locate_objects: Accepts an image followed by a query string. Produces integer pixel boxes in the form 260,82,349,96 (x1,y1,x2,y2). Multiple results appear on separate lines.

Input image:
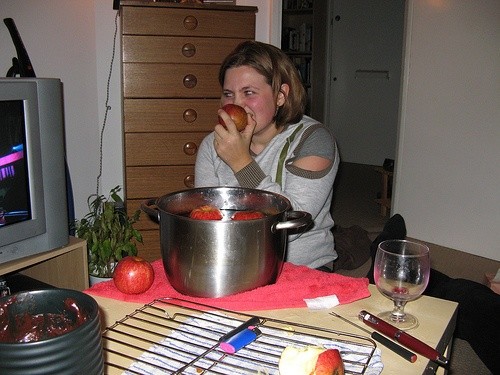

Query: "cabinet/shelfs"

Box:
118,1,259,262
281,0,314,118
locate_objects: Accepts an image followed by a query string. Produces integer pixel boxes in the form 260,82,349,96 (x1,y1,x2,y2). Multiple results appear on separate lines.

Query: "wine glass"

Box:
373,239,430,331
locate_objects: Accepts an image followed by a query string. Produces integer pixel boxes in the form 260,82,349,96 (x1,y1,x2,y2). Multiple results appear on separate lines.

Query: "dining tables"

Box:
56,242,461,375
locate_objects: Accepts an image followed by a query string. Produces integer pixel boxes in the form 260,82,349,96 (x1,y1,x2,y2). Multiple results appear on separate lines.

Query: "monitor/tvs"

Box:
0,77,69,264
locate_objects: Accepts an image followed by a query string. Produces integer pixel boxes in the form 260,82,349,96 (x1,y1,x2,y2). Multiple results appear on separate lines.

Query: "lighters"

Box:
219,324,263,355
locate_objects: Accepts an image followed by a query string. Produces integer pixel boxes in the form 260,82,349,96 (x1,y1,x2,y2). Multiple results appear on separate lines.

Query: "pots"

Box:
141,185,311,297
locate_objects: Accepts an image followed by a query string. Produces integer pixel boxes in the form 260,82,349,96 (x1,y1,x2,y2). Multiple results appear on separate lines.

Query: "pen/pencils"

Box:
358,310,449,367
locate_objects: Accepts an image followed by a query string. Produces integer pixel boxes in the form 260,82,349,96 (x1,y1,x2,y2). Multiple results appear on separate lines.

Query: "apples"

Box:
113,205,265,295
217,104,248,132
277,345,345,375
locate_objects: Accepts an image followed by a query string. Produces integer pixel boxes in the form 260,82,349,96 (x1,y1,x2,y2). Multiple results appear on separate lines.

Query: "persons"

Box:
363,212,500,374
194,40,338,272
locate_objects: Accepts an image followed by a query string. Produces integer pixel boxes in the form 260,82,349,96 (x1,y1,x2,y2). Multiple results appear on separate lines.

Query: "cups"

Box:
0,289,104,375
